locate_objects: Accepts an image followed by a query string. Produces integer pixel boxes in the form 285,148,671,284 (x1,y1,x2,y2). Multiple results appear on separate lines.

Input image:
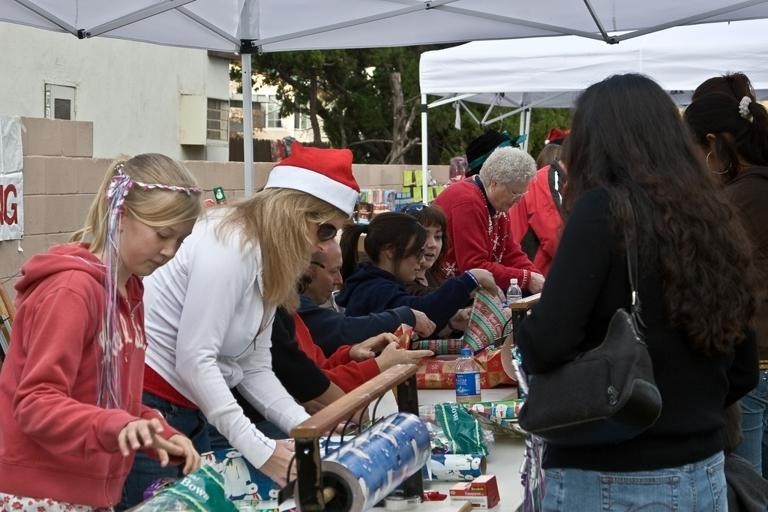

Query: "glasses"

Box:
400,248,425,259
505,183,529,202
316,223,337,242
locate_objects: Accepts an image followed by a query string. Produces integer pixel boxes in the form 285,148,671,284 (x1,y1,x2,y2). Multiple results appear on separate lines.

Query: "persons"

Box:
0,152,204,512
508,134,573,278
297,238,436,357
682,72,768,479
117,140,369,509
431,146,547,293
509,73,762,512
538,137,564,172
293,299,434,394
466,127,525,181
270,303,370,437
335,210,498,338
401,204,472,339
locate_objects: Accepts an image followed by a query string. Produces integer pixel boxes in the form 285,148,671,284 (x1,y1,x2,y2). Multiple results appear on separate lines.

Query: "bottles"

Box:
455,349,481,411
507,277,522,306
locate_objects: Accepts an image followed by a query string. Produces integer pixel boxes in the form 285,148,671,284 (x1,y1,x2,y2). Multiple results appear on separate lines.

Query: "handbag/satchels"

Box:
517,190,663,444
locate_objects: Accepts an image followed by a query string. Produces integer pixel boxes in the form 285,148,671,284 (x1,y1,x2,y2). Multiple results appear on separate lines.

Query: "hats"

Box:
263,141,361,219
465,128,527,178
